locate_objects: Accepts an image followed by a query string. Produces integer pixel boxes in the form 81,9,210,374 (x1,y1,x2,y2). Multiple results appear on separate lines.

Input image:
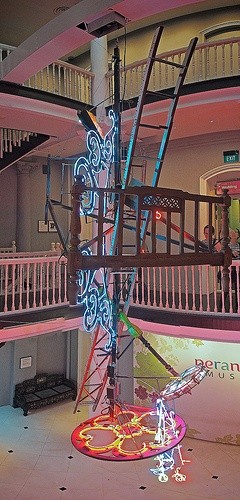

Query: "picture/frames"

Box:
49,220,58,232
20,356,32,369
38,220,48,233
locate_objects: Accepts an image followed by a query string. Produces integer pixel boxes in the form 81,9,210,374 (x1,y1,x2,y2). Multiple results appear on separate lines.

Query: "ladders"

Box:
69,24,198,414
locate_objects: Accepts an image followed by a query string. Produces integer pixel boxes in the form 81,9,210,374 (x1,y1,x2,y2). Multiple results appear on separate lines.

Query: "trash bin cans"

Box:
113,241,151,304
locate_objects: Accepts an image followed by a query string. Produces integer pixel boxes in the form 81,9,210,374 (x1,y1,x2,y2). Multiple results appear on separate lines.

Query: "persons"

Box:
199,224,222,253
216,227,240,313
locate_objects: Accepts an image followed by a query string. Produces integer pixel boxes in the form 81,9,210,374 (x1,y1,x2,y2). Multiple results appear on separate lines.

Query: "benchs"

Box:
12,372,77,416
